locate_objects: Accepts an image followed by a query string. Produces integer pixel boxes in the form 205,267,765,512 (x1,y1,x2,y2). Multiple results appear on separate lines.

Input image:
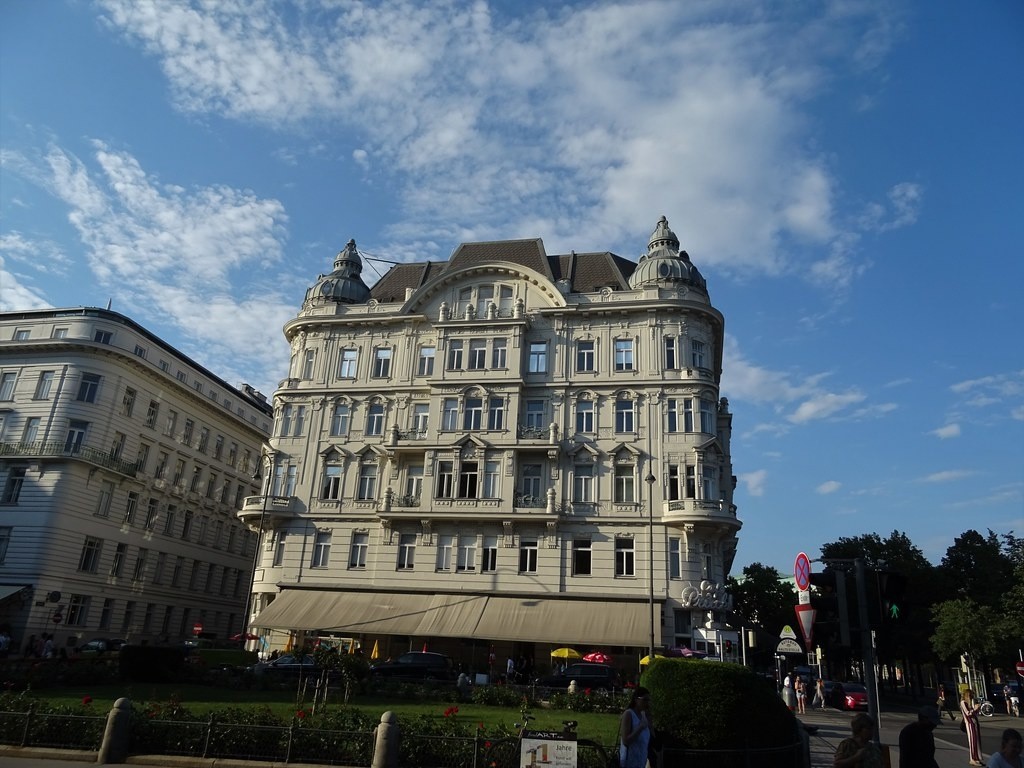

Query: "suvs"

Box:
545,663,624,694
368,652,457,682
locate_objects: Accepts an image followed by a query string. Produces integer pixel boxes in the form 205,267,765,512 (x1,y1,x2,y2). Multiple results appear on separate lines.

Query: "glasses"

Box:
637,697,650,702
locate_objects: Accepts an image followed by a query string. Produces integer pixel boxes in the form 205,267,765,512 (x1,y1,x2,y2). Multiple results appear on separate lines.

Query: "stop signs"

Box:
194,624,203,634
53,614,62,623
1016,660,1024,677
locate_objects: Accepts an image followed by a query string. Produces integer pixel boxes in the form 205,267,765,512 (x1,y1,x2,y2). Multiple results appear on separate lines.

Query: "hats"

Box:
918,706,943,725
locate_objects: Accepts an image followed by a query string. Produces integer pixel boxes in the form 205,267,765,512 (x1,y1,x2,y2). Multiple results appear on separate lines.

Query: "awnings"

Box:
249,590,665,649
0,586,26,599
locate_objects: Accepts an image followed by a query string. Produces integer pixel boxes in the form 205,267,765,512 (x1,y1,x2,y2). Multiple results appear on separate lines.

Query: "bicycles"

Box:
976,697,995,717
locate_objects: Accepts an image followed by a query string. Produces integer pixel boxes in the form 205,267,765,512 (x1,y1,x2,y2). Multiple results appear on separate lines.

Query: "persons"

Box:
271,649,278,660
989,728,1024,768
961,689,983,766
938,684,956,720
0,631,58,658
1003,683,1013,715
899,707,944,768
620,687,652,768
295,645,392,664
568,680,579,694
451,654,530,687
783,673,825,713
833,713,884,768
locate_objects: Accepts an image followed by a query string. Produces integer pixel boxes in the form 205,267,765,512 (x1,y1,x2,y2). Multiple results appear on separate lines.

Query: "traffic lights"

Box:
884,598,903,620
725,639,732,654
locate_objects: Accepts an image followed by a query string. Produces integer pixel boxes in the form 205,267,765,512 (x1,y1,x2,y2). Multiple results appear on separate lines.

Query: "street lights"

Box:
644,468,655,657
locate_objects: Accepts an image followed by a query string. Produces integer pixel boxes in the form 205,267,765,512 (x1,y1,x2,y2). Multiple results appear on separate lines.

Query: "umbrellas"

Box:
489,645,497,685
230,634,260,640
285,635,378,659
640,654,664,665
551,648,612,668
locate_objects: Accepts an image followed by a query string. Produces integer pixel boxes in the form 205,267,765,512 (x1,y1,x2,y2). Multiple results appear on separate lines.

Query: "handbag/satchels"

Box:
960,721,967,733
606,751,626,768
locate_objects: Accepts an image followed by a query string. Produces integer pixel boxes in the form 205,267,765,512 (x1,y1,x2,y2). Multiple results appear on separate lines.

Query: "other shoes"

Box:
970,760,982,766
952,717,956,720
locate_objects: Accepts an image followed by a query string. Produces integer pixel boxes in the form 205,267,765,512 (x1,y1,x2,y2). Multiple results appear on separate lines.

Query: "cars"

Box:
249,652,324,683
828,683,881,711
